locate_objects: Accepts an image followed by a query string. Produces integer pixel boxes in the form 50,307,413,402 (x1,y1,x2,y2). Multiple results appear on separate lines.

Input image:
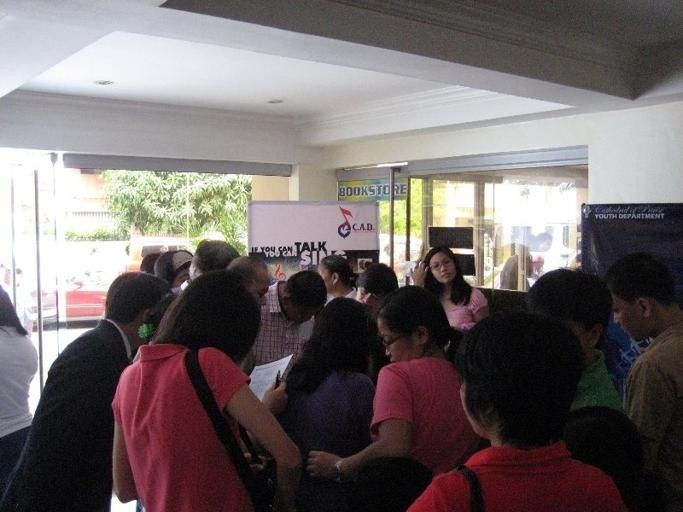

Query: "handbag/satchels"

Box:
246,449,282,510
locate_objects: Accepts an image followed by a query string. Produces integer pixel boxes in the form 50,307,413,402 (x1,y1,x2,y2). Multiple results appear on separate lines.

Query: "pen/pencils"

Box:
275,370,280,389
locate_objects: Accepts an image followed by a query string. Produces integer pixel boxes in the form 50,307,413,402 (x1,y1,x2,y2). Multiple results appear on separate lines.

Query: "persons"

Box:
1,283,42,487
522,267,626,414
109,269,305,511
1,269,178,510
547,403,644,492
139,239,490,484
605,249,682,510
402,306,629,510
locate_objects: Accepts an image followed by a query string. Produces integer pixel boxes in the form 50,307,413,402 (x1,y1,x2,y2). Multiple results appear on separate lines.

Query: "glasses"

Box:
382,334,405,347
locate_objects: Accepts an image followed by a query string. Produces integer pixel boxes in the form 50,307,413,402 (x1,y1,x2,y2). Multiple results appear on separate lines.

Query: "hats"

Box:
155,250,194,278
